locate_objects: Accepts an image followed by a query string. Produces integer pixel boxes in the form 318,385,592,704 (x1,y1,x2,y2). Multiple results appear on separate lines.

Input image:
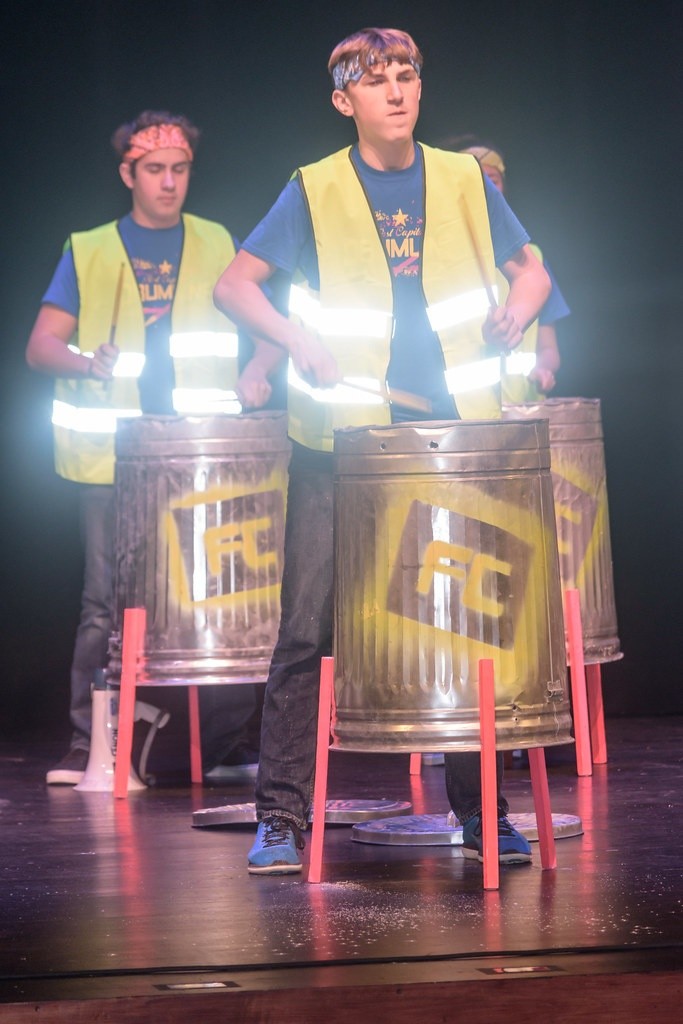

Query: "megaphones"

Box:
72,668,169,792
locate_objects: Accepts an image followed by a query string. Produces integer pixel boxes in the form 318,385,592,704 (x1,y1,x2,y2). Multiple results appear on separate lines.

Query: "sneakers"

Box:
247,815,305,875
462,810,532,864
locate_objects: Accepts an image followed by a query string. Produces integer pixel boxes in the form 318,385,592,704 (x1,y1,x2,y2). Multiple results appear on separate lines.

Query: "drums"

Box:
499,395,625,668
103,408,293,688
326,415,577,755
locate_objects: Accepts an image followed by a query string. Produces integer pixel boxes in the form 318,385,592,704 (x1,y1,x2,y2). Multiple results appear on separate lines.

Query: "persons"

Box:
25,112,285,783
460,147,571,394
212,26,551,875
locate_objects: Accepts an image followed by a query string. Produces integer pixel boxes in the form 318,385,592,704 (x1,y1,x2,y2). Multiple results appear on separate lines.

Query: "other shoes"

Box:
512,750,522,758
204,741,259,780
421,752,445,766
46,748,90,784
